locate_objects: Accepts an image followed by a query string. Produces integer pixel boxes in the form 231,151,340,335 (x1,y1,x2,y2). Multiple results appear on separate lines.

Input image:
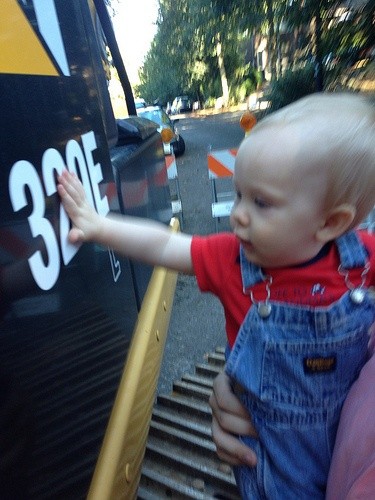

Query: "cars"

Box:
135,99,186,158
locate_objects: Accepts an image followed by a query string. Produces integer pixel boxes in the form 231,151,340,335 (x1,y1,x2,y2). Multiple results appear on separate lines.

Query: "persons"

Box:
59,91,375,500
211,353,375,500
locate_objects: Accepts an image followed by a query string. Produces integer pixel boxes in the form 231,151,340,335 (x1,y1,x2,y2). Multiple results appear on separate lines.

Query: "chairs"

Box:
207,144,239,233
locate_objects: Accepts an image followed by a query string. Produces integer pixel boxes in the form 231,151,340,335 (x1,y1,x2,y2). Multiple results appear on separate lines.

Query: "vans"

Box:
170,95,193,114
